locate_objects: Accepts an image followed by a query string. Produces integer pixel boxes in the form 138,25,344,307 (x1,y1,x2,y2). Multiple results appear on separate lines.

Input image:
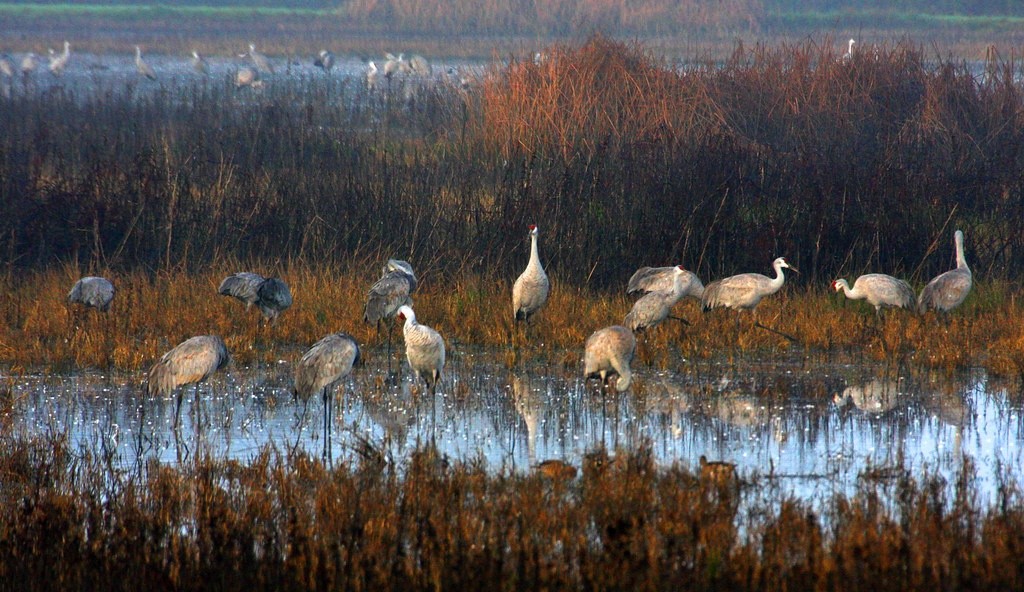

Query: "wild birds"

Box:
236,44,434,96
148,335,229,425
843,39,855,61
134,45,158,81
626,267,706,301
584,326,637,392
513,374,970,453
0,41,71,78
191,50,209,77
830,273,917,322
699,257,803,341
623,265,694,344
917,230,972,328
66,277,115,313
217,272,293,322
294,332,360,435
396,304,445,400
513,224,549,333
361,258,418,342
